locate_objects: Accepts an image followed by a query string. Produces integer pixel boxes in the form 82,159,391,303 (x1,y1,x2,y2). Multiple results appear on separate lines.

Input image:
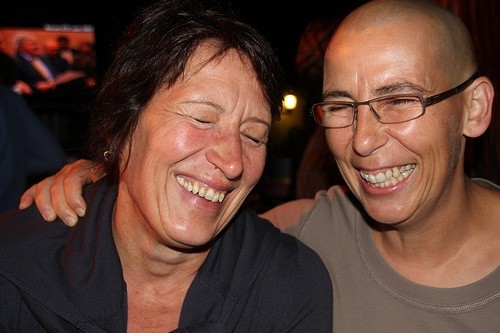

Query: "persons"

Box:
17,0,500,333
0,0,333,333
0,30,95,212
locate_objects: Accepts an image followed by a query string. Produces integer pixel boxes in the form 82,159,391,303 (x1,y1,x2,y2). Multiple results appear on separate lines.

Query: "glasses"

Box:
309,72,477,130
